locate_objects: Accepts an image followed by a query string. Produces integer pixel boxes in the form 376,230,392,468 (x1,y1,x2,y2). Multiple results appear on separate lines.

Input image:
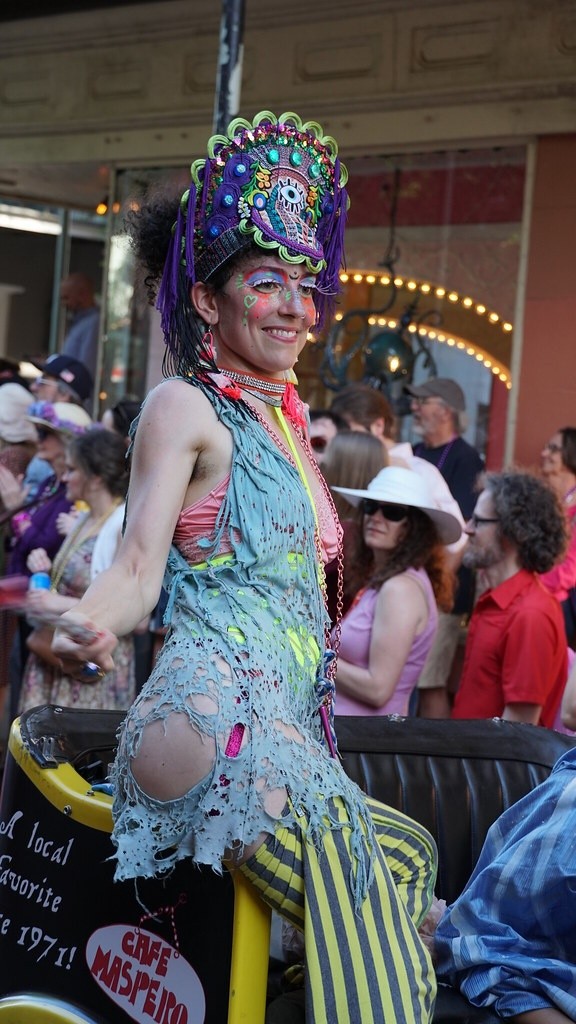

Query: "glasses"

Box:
359,499,411,523
36,429,57,440
35,376,60,389
310,437,327,453
406,395,445,406
544,443,562,453
471,513,501,528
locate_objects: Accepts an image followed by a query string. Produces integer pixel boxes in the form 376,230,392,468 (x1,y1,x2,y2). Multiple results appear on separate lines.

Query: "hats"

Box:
26,401,93,438
0,383,39,443
403,378,466,412
25,353,93,399
330,466,462,547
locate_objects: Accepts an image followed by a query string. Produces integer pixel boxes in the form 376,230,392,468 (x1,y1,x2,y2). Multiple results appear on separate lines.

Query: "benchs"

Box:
1,702,575,1023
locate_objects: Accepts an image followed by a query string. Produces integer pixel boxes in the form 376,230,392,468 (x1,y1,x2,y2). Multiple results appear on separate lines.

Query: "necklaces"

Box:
239,396,344,717
51,497,123,592
414,437,457,474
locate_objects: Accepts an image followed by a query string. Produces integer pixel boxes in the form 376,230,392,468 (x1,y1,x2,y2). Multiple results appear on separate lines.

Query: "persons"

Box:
409,378,485,716
19,428,136,713
539,426,576,645
433,745,576,1024
329,472,441,716
450,466,570,731
0,353,94,775
49,188,441,1024
307,384,469,568
